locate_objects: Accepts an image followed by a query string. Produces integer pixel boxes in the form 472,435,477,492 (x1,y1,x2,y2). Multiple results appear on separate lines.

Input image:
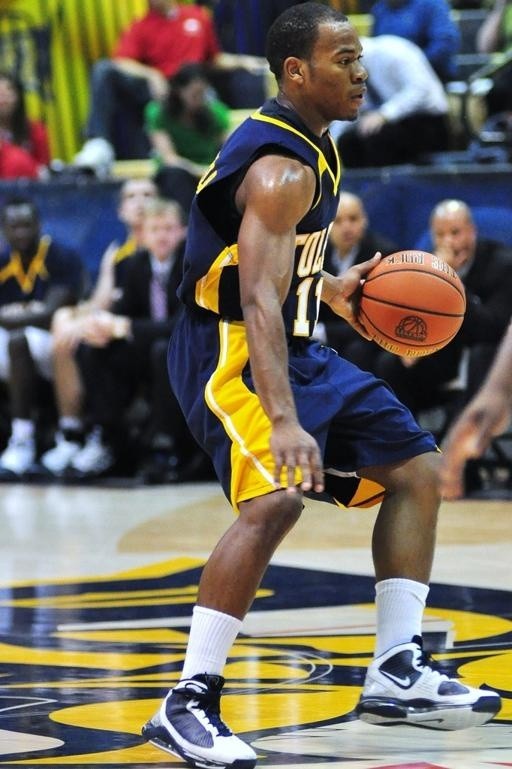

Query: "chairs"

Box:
116,0,510,172
408,204,510,490
78,215,125,278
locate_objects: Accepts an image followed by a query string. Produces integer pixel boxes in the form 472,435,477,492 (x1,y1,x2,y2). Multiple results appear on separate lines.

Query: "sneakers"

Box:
353,634,502,730
141,672,259,769
71,439,113,474
42,430,80,474
0,436,35,474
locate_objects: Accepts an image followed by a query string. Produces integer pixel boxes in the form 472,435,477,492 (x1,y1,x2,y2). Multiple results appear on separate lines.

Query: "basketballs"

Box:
358,248,466,359
